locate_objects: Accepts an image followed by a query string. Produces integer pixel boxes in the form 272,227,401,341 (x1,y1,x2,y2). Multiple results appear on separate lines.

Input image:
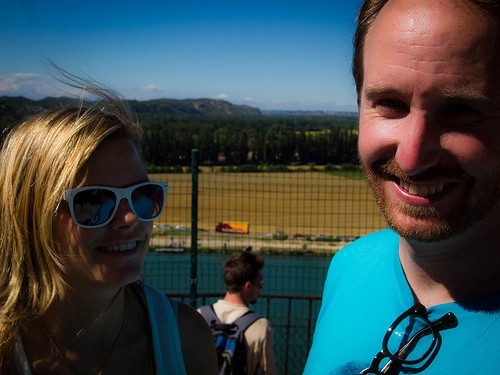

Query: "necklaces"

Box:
98,286,127,375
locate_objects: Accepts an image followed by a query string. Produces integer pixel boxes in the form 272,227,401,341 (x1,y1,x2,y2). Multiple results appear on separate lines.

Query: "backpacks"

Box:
198,305,263,375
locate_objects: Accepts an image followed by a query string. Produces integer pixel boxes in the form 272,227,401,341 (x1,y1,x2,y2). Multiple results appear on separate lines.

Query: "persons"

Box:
300,0,500,375
0,107,188,375
196,252,273,375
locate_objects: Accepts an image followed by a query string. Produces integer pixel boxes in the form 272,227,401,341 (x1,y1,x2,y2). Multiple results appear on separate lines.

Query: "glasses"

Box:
59,181,173,228
357,299,458,375
